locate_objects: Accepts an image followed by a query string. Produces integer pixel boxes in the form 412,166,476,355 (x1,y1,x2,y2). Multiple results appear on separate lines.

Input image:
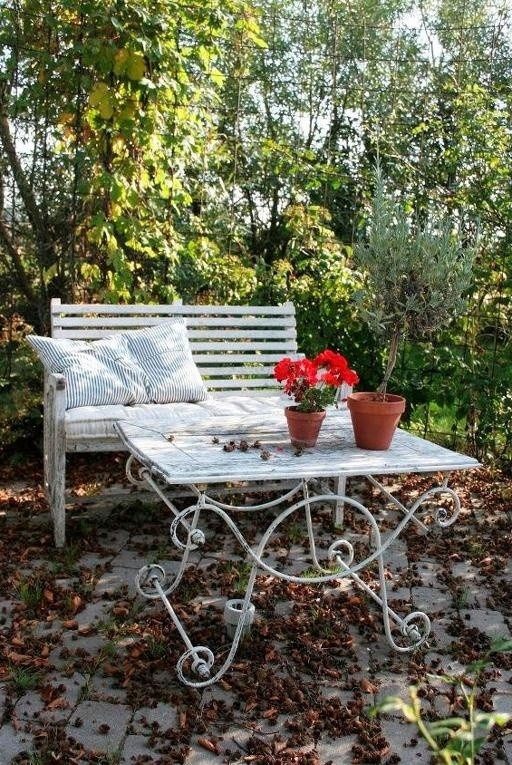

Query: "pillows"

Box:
24,317,210,411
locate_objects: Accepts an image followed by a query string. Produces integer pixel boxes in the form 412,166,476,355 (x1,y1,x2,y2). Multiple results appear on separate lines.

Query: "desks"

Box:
107,419,487,689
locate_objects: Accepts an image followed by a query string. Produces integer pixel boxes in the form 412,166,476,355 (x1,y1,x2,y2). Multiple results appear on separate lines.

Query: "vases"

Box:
284,405,327,448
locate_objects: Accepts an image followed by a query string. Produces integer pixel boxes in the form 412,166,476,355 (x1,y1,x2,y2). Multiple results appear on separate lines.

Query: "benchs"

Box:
41,295,360,549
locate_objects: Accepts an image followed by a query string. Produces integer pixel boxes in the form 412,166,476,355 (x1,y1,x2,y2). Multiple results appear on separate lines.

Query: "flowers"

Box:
275,347,362,413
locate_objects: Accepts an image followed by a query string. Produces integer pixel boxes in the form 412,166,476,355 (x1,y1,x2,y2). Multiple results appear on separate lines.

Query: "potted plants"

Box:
343,114,477,450
